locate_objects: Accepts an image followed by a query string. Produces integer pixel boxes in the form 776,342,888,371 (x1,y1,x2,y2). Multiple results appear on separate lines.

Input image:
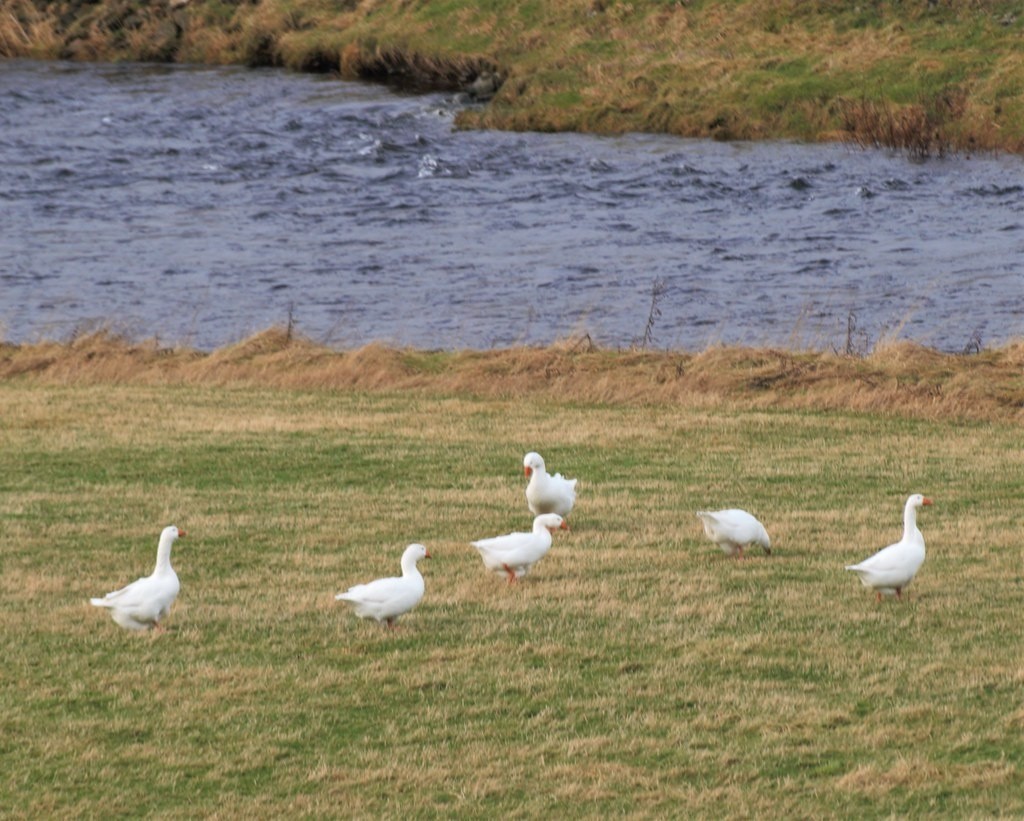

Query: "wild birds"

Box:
469,512,569,586
337,544,433,631
524,451,578,521
86,525,187,631
845,494,932,603
698,507,773,558
